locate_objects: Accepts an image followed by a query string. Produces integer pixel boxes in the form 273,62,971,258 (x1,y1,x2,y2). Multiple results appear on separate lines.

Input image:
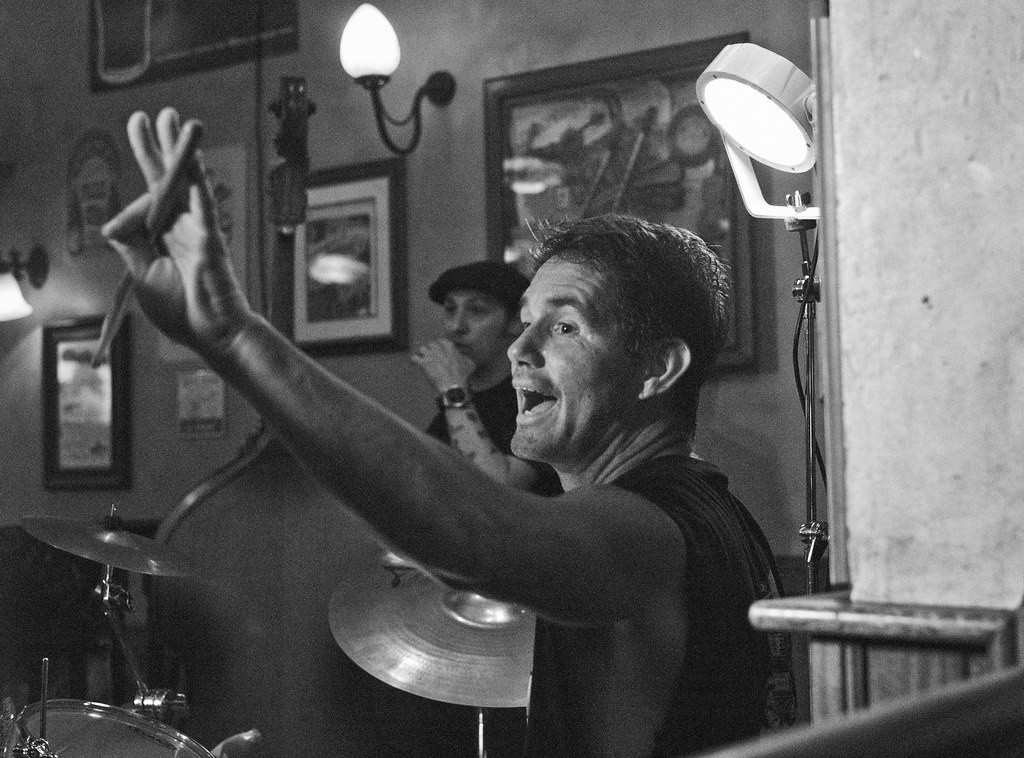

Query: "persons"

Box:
100,106,797,758
411,260,564,497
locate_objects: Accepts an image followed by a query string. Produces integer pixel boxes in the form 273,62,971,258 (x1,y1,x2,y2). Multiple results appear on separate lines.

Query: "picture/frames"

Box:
40,312,129,490
270,160,408,358
482,31,757,371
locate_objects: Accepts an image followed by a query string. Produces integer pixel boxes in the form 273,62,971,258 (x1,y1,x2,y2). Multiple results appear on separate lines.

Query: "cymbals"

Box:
328,547,536,708
19,514,205,579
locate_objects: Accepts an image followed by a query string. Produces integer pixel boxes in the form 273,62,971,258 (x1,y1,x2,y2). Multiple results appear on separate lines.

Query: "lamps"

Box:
695,43,821,220
339,3,455,153
0,242,48,321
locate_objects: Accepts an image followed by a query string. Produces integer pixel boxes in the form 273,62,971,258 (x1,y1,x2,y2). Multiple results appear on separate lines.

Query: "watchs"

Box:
436,383,475,411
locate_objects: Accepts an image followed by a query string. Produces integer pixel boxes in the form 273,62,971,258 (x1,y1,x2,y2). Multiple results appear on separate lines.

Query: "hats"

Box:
429,261,530,314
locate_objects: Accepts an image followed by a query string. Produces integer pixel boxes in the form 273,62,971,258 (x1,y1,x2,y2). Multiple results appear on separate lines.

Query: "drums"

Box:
1,690,218,758
